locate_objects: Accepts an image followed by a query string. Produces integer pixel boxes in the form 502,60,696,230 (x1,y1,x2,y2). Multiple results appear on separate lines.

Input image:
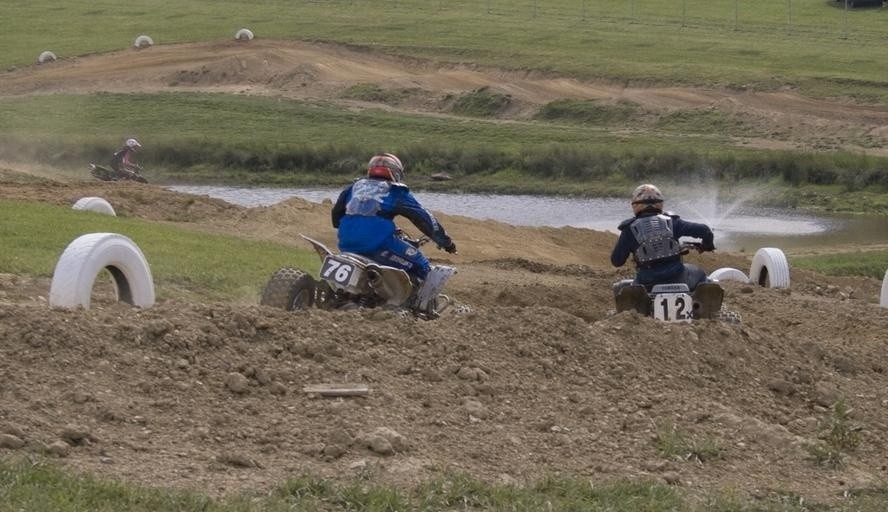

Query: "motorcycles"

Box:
91,161,148,185
256,230,459,321
610,241,740,333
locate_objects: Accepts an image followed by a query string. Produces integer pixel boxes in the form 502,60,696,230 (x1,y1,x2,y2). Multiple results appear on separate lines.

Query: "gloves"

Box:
701,241,713,253
444,242,456,254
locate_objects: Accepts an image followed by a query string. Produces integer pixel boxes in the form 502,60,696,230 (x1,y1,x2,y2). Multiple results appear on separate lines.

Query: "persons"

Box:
107,137,144,182
610,181,716,293
329,151,457,290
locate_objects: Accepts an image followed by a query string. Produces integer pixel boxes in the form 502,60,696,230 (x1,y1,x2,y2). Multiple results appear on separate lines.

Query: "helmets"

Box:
630,183,665,218
366,152,404,183
